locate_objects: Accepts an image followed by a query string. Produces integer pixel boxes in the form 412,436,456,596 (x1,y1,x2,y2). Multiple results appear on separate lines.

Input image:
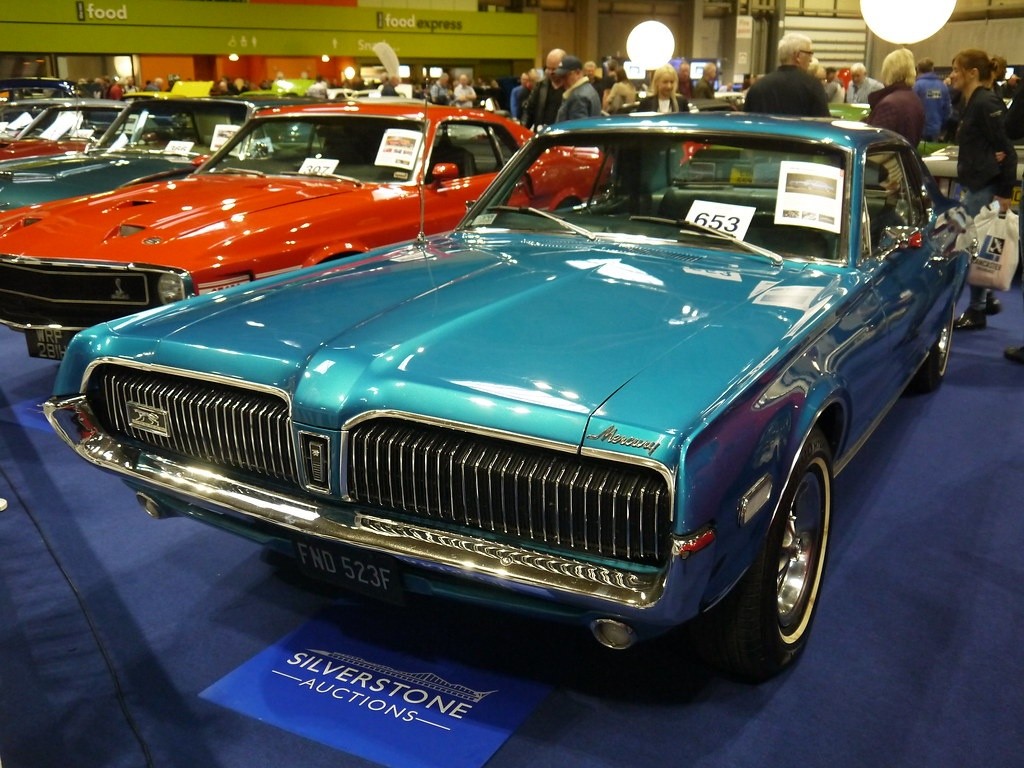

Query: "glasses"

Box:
800,50,814,55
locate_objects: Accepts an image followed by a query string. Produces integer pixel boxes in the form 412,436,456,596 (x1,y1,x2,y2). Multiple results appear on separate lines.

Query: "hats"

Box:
551,57,582,76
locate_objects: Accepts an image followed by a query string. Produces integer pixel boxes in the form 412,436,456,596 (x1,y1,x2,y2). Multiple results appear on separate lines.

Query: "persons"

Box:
861,47,926,151
375,70,506,111
765,58,1024,142
509,48,695,134
258,70,368,101
695,45,1024,151
76,75,250,99
742,31,831,118
947,48,1019,331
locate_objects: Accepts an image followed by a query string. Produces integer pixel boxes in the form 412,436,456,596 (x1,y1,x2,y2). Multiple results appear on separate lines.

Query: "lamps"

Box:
626,20,675,71
860,0,957,46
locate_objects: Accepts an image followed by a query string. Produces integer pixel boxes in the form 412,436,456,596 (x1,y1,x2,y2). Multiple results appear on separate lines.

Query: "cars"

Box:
0,98,346,211
40,111,980,678
0,103,710,362
0,98,127,161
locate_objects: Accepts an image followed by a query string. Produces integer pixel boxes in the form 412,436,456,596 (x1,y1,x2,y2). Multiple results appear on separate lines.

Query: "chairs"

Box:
428,147,477,184
320,131,376,166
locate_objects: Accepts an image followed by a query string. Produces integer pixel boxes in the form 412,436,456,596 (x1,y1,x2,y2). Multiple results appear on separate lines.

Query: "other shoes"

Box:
1005,346,1024,361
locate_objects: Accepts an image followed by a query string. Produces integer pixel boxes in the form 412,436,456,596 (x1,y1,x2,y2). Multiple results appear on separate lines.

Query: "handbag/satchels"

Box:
966,202,1019,292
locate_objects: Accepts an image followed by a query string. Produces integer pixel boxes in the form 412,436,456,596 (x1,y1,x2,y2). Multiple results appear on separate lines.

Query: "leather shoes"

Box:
953,311,987,330
985,298,1002,315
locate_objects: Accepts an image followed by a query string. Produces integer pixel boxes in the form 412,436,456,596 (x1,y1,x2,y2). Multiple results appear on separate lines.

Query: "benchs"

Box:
370,144,510,176
627,188,915,251
198,142,321,158
